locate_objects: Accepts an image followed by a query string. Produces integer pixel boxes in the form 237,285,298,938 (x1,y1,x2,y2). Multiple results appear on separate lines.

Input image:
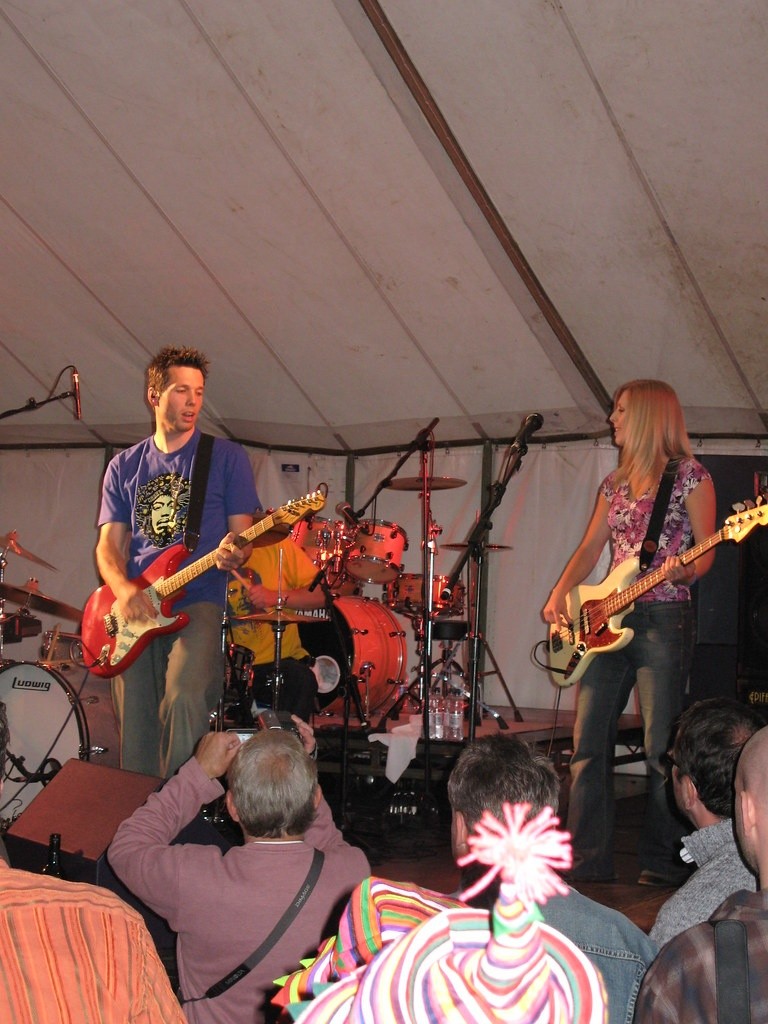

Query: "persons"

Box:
106,714,374,1024
225,515,331,722
540,380,718,888
96,345,256,777
0,846,190,1023
440,696,768,1024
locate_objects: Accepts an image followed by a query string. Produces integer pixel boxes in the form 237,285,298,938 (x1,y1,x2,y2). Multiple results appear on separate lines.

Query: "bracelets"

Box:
684,575,696,587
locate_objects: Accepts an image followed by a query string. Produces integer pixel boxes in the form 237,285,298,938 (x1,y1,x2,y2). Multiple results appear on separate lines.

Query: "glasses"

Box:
659,751,700,793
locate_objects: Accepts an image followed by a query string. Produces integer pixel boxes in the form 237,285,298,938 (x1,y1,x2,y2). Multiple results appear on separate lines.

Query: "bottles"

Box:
428,687,445,739
446,689,455,739
450,689,465,740
395,671,408,712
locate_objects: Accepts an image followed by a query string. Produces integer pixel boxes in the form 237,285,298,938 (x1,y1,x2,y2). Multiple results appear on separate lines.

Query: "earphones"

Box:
151,395,155,399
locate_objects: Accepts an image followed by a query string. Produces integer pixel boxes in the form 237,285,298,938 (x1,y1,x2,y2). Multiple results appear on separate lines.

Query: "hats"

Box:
273,800,610,1024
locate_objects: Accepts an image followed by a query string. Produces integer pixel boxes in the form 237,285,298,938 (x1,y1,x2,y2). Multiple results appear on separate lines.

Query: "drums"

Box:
225,642,254,698
299,595,408,717
343,518,407,585
289,515,362,595
387,572,463,617
0,663,119,828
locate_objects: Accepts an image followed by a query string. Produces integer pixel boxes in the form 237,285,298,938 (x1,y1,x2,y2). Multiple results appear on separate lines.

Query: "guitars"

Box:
82,489,324,679
549,494,768,687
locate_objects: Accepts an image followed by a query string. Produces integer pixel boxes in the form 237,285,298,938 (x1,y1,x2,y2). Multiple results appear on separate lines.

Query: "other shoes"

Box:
638,865,693,888
556,856,619,882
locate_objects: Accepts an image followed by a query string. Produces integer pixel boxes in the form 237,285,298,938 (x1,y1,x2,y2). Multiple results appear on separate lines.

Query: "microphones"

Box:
335,501,370,535
509,413,545,455
71,367,81,420
14,769,58,783
308,556,336,593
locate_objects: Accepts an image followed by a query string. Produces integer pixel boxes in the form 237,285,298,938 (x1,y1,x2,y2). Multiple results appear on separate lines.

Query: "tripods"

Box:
432,558,524,727
320,574,387,859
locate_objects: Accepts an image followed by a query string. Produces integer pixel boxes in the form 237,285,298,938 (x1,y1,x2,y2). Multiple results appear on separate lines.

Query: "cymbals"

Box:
441,543,511,554
230,611,326,627
1,538,58,573
0,582,83,621
385,475,468,492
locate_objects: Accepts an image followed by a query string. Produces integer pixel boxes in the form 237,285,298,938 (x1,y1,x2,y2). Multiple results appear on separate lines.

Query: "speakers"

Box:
1,758,233,946
687,453,768,725
